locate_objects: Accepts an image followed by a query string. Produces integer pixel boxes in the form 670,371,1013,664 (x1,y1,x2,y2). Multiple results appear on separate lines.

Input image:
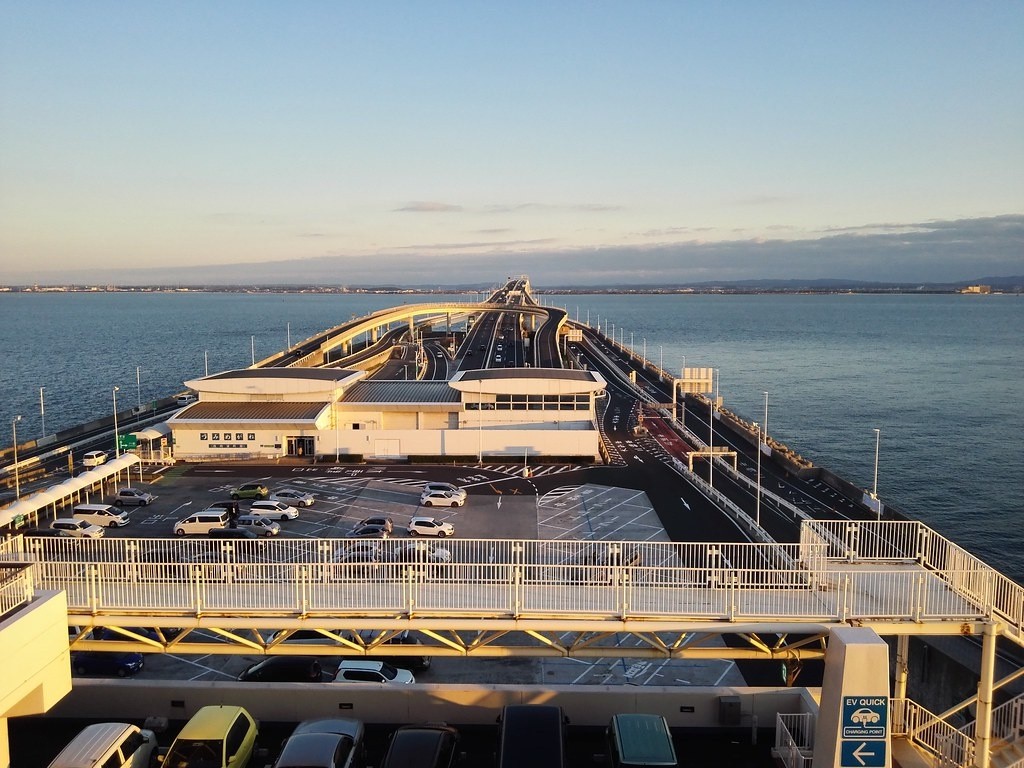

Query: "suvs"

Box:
81,450,108,468
603,712,678,768
492,704,570,768
155,705,260,768
45,722,160,768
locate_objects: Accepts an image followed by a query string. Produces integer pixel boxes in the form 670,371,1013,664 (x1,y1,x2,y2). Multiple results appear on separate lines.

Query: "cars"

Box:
377,721,467,768
437,312,516,367
272,715,367,768
17,478,468,683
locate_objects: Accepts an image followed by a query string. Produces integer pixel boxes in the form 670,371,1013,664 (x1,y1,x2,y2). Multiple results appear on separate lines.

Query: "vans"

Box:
177,395,197,406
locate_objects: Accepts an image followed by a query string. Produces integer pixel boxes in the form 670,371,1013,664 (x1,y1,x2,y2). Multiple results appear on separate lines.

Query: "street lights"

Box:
702,397,713,495
871,427,881,497
750,422,761,537
596,314,600,335
630,332,634,361
250,334,255,364
11,414,22,501
420,330,424,362
112,386,120,459
643,338,646,369
414,350,418,379
403,364,408,381
762,391,769,445
605,319,608,340
204,351,209,376
287,321,291,350
619,328,623,353
611,324,615,346
681,356,686,368
869,493,881,557
658,345,663,382
136,365,141,406
714,369,719,412
40,386,46,438
